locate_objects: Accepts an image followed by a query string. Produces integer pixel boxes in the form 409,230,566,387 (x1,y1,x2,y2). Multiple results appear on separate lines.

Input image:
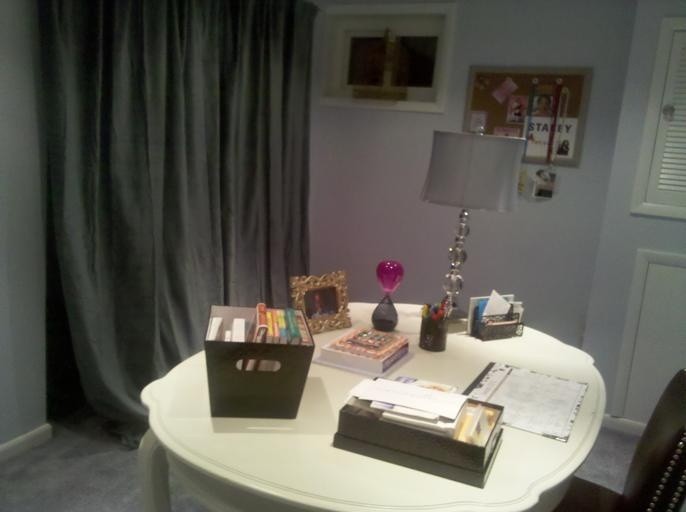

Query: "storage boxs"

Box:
204,304,315,419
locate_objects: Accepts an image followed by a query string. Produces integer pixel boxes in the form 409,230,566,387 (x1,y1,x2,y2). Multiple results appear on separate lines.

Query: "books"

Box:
337,371,506,488
314,325,411,378
205,299,314,373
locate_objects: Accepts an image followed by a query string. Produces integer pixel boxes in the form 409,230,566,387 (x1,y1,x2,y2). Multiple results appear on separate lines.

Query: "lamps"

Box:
419,130,526,322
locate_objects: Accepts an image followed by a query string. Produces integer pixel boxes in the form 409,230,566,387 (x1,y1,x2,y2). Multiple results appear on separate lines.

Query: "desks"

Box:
139,301,606,512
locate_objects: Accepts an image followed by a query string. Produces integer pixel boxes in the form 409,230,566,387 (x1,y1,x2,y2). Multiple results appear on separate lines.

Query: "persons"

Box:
309,290,334,316
533,95,553,117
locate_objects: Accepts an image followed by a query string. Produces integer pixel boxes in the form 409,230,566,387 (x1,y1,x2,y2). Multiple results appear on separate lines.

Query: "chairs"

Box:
551,368,686,512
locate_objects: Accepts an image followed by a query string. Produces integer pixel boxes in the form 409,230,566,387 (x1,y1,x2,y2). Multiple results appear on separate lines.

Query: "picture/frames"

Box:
290,269,352,334
